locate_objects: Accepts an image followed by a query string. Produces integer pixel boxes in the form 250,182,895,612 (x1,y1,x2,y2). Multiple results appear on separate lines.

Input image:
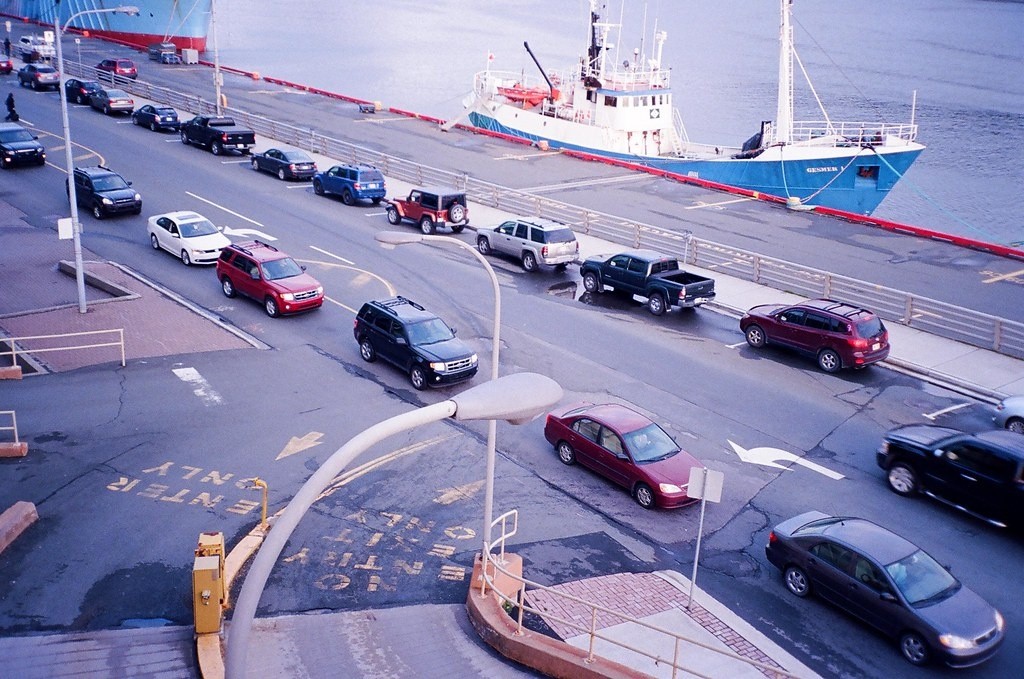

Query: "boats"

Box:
0,0,213,53
466,1,926,217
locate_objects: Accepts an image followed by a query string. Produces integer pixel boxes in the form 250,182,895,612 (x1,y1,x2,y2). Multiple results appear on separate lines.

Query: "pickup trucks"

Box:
578,250,716,315
179,115,255,156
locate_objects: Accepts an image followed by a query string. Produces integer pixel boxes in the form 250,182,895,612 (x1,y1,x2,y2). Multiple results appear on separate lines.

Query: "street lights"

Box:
374,229,502,566
54,4,141,313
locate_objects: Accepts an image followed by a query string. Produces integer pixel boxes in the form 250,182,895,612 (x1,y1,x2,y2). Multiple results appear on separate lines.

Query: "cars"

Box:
147,210,232,268
0,123,47,169
991,394,1023,436
132,104,181,132
544,401,708,511
16,64,60,90
60,79,102,103
90,89,133,115
93,57,139,81
0,54,13,76
312,165,390,205
251,147,317,182
764,508,1006,670
876,421,1024,539
18,36,57,60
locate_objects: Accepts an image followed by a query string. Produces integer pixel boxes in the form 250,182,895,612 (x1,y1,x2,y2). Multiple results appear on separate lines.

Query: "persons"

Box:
3,39,12,57
31,49,40,63
5,93,15,123
889,561,912,589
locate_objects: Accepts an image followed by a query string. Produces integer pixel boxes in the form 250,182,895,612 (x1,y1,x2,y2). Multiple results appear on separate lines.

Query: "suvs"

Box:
217,240,324,317
67,165,143,218
474,216,581,273
387,186,469,236
739,297,891,372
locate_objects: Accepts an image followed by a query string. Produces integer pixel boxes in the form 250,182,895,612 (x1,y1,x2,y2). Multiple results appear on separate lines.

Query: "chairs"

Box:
848,551,858,577
591,422,601,441
602,428,622,455
